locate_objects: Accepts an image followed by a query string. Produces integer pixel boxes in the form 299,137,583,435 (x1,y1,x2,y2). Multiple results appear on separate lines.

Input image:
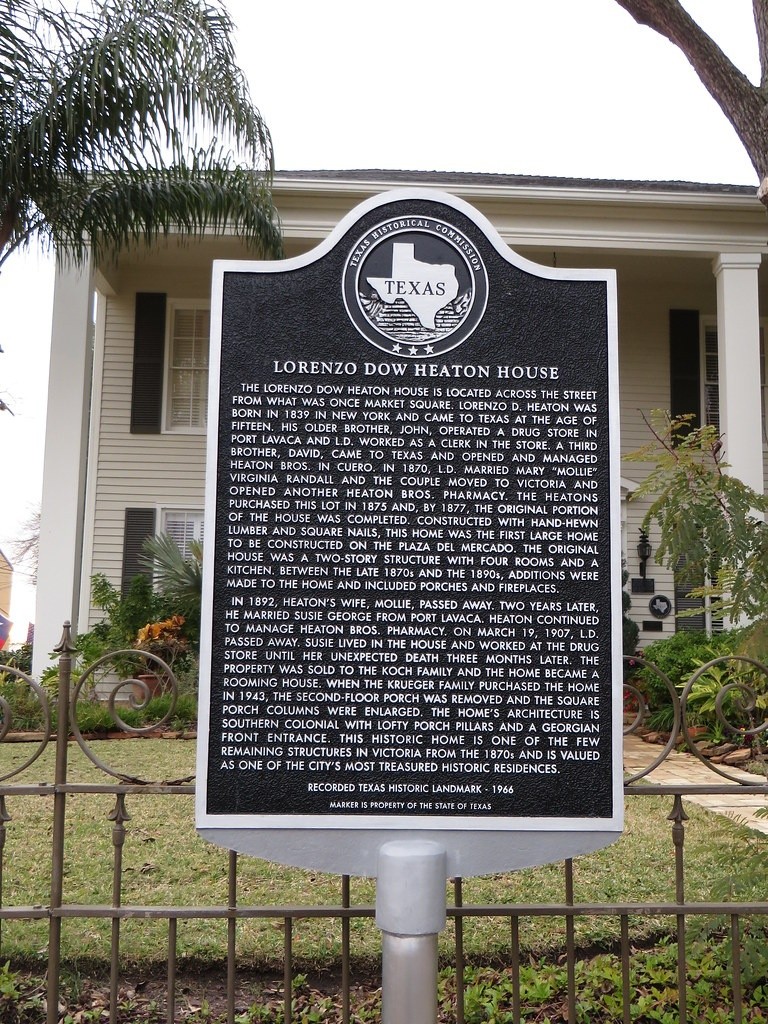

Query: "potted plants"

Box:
686,713,710,738
131,614,188,701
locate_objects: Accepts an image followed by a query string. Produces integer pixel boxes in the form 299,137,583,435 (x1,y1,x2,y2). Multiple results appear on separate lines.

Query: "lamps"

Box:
637,527,652,579
